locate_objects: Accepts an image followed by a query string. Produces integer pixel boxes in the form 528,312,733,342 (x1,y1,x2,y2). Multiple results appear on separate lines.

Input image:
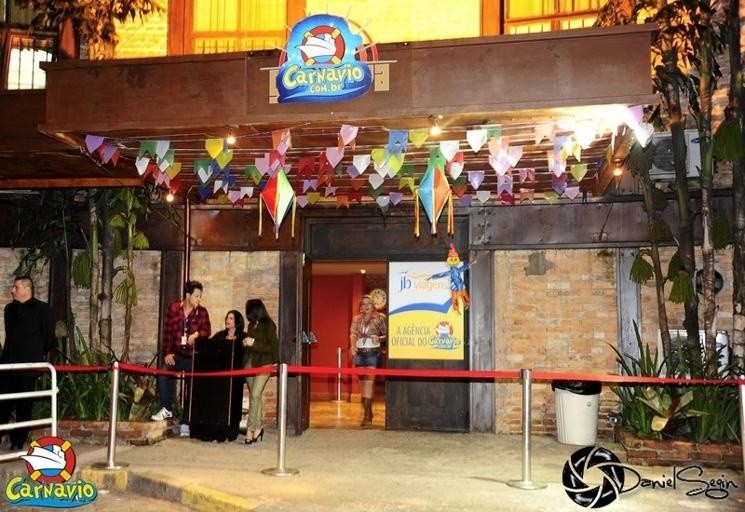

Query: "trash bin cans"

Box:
551,380,602,446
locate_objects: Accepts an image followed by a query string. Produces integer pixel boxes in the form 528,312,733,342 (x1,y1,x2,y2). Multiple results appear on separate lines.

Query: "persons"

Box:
150,279,212,437
0,275,57,451
206,309,249,342
350,294,387,426
239,298,278,444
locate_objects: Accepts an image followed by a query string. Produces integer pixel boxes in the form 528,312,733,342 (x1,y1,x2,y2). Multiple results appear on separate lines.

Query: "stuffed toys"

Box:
425,243,479,315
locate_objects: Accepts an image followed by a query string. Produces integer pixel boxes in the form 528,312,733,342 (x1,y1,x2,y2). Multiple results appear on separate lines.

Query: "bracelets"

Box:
376,335,381,342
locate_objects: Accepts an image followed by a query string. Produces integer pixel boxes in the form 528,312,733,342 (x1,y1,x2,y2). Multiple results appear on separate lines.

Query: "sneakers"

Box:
180,423,190,438
151,407,173,421
10,443,23,452
245,434,253,444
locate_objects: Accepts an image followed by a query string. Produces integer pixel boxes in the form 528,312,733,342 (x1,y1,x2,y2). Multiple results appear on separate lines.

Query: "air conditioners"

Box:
641,130,702,179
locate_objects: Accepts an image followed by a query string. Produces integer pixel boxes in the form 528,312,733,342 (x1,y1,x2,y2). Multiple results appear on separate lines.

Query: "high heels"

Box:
252,427,264,442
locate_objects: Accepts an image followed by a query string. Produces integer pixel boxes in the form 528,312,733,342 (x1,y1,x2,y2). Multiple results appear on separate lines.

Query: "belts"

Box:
357,348,378,354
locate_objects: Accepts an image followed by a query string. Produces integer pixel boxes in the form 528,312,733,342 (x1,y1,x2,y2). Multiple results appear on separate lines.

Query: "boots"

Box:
361,397,373,427
